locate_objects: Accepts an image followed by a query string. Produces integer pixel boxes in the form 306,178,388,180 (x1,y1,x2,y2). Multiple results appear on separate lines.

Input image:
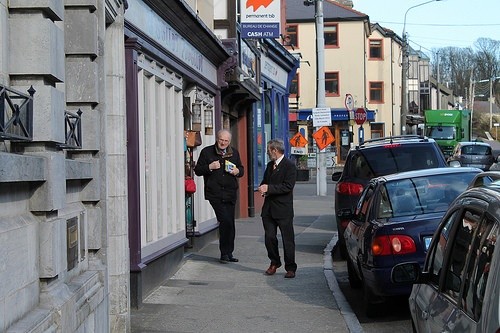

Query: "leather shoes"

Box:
220,254,239,262
284,270,296,278
265,264,282,275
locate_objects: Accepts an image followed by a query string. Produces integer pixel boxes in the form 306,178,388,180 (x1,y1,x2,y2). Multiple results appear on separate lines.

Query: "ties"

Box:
272,163,277,171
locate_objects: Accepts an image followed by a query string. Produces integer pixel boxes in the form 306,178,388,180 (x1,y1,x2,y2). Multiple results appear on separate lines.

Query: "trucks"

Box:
421,109,471,161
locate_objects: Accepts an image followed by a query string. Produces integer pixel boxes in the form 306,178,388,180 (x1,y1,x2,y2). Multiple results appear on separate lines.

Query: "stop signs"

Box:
354,107,367,125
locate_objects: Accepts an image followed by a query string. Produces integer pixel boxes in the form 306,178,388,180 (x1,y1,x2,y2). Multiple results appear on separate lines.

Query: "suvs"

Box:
390,169,500,333
317,134,450,261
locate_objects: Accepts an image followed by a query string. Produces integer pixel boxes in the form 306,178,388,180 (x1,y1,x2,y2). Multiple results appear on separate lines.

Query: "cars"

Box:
446,141,495,171
331,166,496,320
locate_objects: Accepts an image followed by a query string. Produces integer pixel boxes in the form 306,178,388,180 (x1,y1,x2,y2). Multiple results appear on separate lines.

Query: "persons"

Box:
259,139,298,278
194,128,245,262
